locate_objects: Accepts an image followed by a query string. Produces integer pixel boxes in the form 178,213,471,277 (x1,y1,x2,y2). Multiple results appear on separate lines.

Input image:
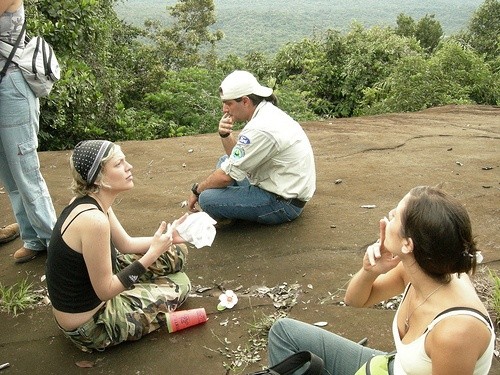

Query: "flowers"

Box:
216,290,238,311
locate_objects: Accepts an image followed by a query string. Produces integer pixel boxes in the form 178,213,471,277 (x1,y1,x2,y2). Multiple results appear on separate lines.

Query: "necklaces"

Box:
404,281,446,335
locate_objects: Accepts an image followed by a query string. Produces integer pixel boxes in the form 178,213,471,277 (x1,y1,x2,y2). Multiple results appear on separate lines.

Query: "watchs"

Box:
191,183,200,198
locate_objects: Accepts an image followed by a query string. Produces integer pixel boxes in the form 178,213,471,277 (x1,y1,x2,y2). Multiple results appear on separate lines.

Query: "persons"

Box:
266,186,495,375
187,69,317,224
45,140,193,353
0,1,60,263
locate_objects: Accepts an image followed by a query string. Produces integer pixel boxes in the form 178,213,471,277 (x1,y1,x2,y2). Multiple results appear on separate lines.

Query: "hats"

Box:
219,70,273,100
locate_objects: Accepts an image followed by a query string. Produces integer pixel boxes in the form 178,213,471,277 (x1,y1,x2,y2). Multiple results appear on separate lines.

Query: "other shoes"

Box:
0,223,19,242
14,246,39,262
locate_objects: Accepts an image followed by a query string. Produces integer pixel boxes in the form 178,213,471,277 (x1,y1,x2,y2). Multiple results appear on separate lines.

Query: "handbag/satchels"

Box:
0,32,60,97
355,354,394,375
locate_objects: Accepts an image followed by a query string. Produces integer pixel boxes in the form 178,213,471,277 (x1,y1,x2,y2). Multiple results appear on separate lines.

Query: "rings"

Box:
377,239,381,244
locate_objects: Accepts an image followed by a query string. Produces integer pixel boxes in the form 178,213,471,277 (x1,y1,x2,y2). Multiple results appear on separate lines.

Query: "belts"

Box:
276,194,307,208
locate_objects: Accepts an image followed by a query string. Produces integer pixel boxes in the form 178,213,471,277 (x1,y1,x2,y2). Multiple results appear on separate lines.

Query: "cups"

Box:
166,307,207,334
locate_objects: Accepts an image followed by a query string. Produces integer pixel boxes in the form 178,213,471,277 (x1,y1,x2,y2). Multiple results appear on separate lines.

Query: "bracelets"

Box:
218,132,230,138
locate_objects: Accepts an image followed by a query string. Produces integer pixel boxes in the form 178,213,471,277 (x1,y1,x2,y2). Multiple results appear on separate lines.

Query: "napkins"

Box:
174,209,222,248
